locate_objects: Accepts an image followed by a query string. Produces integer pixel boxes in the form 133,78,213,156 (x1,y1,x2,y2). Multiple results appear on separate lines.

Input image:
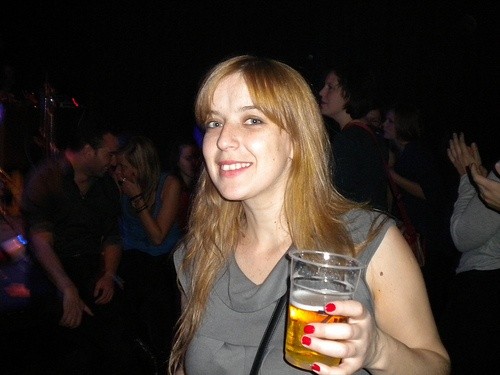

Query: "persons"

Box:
166,55,451,375
0,61,500,375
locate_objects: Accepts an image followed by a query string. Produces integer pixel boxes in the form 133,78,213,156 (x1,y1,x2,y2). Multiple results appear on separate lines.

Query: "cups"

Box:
284,251,363,372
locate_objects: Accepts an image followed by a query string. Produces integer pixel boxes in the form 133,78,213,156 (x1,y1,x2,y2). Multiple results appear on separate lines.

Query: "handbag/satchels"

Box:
392,218,425,267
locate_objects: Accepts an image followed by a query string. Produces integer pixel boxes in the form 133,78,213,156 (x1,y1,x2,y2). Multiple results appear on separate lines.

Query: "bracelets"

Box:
127,192,148,214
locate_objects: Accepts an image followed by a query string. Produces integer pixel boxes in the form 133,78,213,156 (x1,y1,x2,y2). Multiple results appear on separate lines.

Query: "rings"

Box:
121,177,127,182
